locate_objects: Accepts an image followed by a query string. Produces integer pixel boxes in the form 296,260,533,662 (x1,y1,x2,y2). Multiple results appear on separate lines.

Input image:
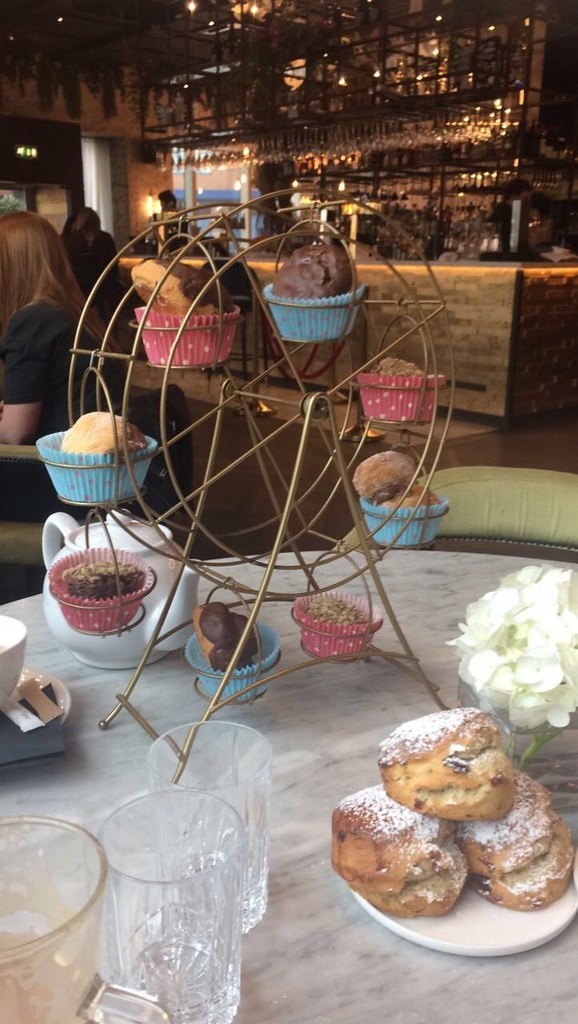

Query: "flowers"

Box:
445,563,578,770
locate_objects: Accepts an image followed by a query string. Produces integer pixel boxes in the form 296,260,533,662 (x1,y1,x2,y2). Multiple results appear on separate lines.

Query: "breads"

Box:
330,707,575,918
58,411,146,456
353,451,439,507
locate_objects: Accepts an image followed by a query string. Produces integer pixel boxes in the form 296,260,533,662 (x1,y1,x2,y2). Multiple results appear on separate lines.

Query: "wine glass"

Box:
154,108,516,169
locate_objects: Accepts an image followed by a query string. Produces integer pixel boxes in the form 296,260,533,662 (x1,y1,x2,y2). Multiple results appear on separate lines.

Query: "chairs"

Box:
0,384,193,605
333,467,578,563
203,260,271,388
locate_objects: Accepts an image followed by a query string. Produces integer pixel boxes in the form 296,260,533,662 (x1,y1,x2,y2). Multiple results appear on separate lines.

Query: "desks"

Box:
0,550,578,1024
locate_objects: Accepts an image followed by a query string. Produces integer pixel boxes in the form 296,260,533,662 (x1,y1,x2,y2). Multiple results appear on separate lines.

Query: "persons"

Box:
490,178,532,260
0,210,128,448
61,207,121,320
157,189,188,252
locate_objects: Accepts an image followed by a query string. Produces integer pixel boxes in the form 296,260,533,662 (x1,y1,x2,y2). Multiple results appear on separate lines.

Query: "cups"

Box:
145,721,272,934
99,790,248,1024
0,815,170,1024
0,615,28,710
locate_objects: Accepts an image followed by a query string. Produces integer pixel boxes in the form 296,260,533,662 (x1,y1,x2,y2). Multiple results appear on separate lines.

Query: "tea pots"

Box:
40,508,208,670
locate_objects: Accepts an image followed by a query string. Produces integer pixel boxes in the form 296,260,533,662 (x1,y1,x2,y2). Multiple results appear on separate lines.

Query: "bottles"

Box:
301,196,503,259
455,168,567,193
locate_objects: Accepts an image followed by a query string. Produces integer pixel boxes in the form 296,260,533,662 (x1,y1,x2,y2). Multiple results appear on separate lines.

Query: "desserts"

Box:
270,244,353,299
192,601,257,673
129,258,232,315
61,559,142,601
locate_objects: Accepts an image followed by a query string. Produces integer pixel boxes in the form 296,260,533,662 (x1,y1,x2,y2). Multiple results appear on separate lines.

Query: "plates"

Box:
348,876,578,956
8,667,72,725
